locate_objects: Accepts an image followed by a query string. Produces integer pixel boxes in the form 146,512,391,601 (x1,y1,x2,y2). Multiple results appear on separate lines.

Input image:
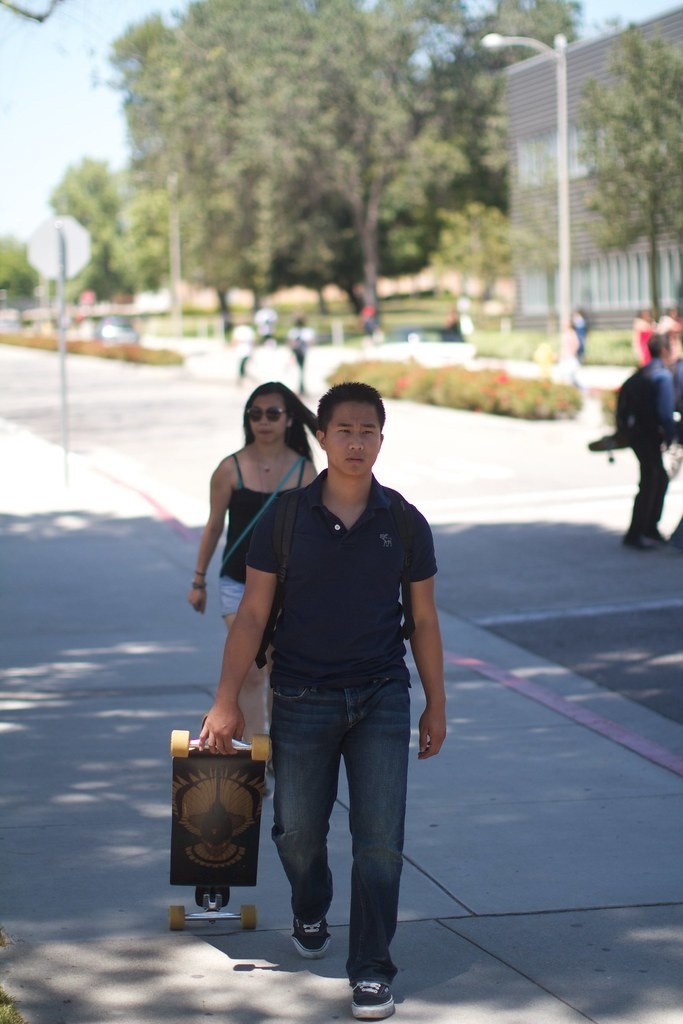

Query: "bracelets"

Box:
195,572,205,576
194,584,206,588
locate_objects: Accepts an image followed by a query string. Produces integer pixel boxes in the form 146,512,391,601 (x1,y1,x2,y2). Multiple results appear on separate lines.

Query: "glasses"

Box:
247,406,287,422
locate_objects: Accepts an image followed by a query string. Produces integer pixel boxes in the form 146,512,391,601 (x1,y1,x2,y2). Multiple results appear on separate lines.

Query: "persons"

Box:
632,311,653,368
288,313,315,363
656,307,683,364
199,384,447,1018
668,330,683,447
253,302,278,339
574,308,590,355
189,384,318,628
234,321,256,360
616,334,676,549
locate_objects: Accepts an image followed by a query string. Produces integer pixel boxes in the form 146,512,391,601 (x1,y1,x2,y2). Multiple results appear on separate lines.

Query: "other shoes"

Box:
646,527,666,542
623,534,650,549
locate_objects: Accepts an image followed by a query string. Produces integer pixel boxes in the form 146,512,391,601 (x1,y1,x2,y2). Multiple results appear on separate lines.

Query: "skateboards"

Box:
165,727,271,932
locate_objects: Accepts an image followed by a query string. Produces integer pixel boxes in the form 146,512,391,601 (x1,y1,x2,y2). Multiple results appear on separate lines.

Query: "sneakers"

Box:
291,914,333,957
351,980,394,1019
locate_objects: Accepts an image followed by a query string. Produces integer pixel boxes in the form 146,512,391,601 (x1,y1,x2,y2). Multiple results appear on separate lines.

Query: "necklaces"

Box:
253,448,280,471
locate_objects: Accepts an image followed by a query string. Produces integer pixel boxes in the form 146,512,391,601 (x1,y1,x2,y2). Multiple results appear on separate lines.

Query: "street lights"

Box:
475,31,571,382
